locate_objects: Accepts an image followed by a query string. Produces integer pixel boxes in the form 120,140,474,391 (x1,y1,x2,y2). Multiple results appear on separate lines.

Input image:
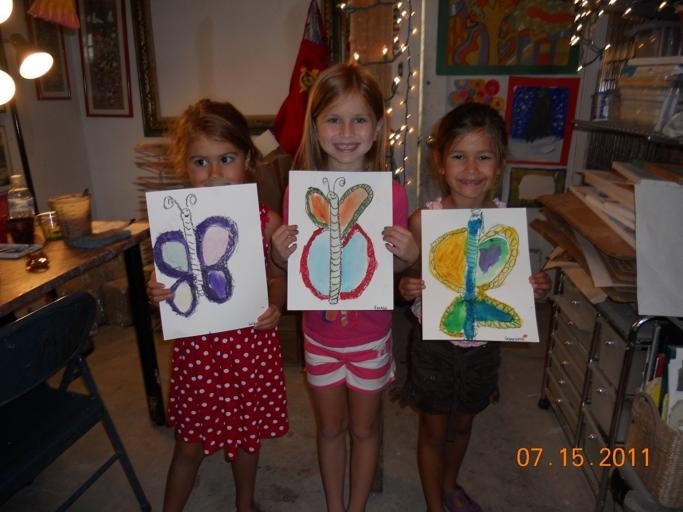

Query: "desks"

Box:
0,221,168,428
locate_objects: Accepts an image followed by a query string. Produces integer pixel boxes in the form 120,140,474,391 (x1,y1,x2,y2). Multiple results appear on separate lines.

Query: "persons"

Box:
397,102,552,511
164,96,287,512
265,62,420,511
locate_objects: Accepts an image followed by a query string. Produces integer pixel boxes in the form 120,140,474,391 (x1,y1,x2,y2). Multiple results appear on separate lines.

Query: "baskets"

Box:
624,391,682,509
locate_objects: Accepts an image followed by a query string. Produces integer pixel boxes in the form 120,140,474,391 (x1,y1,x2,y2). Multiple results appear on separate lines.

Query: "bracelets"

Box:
270,255,285,271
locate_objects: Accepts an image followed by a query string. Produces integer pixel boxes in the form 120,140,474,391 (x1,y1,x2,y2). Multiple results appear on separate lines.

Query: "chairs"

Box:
0,293,154,512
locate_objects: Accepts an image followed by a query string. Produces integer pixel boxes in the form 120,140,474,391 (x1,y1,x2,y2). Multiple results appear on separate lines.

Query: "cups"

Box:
0,173,94,245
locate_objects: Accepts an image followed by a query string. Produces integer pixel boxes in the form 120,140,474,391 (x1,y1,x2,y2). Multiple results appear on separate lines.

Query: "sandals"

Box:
443,484,482,512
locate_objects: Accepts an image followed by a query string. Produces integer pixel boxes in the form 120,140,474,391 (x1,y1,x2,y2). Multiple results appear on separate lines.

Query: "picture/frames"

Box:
436,0,593,208
76,0,135,118
129,0,342,137
23,0,73,100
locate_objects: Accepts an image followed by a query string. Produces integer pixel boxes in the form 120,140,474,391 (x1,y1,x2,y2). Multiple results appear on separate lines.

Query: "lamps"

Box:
0,0,55,216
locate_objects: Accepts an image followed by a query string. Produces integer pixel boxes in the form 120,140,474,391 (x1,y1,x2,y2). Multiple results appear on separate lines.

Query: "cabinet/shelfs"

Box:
539,263,667,512
